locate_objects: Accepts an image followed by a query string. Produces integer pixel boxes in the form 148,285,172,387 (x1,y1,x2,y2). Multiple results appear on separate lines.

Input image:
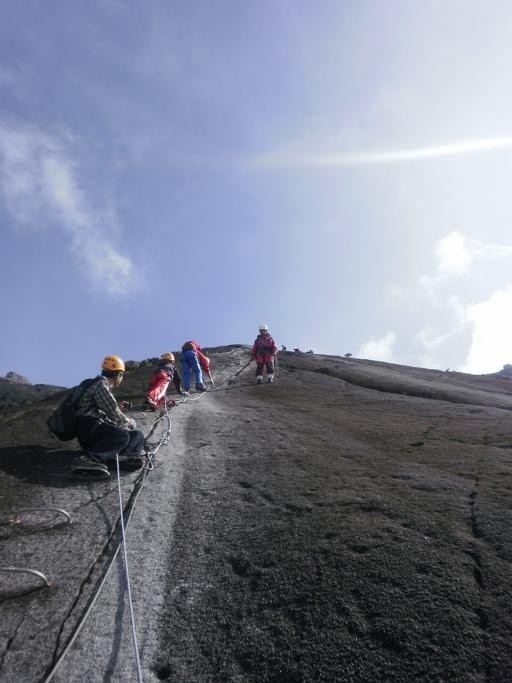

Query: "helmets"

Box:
162,352,175,361
101,355,126,372
259,324,268,330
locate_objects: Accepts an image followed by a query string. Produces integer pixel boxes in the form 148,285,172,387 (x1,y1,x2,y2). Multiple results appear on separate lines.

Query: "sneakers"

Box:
107,453,144,472
70,455,111,477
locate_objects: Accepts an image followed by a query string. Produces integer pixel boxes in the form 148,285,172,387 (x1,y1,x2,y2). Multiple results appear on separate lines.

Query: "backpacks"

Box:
47,376,107,441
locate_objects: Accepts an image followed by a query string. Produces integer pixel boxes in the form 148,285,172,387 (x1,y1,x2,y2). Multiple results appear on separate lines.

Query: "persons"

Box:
251,324,276,384
72,355,142,475
180,341,214,394
145,352,181,410
